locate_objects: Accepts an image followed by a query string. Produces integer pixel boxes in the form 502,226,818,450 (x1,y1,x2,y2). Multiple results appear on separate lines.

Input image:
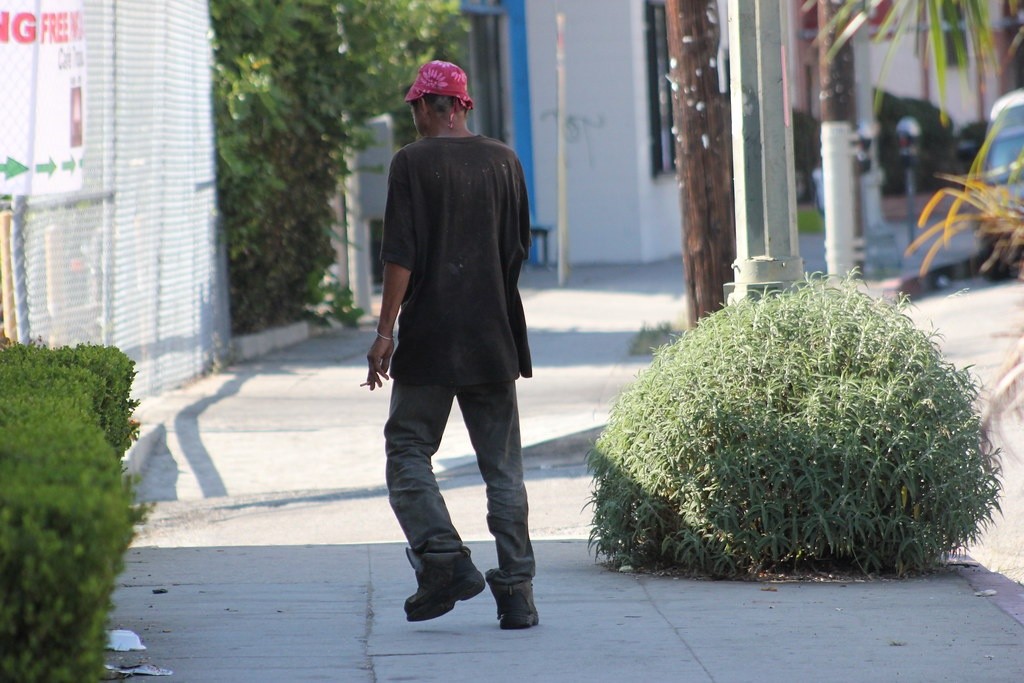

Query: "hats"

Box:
404,60,473,109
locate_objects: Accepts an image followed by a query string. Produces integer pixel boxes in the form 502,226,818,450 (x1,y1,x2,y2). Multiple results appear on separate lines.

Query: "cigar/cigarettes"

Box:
360,382,370,387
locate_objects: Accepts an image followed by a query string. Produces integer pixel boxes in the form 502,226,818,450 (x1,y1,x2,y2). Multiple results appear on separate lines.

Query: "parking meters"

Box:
897,114,923,256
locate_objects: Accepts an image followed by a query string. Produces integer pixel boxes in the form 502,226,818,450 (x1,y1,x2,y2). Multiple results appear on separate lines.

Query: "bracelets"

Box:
374,328,394,340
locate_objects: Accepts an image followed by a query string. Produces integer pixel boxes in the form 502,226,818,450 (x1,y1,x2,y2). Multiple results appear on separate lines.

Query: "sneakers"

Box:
404,553,484,621
489,577,540,629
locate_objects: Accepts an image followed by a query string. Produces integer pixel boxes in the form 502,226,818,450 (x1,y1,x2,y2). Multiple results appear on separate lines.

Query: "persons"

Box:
365,60,541,628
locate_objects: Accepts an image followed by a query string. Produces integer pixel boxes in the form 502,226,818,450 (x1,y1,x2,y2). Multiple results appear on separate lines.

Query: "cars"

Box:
964,85,1024,280
